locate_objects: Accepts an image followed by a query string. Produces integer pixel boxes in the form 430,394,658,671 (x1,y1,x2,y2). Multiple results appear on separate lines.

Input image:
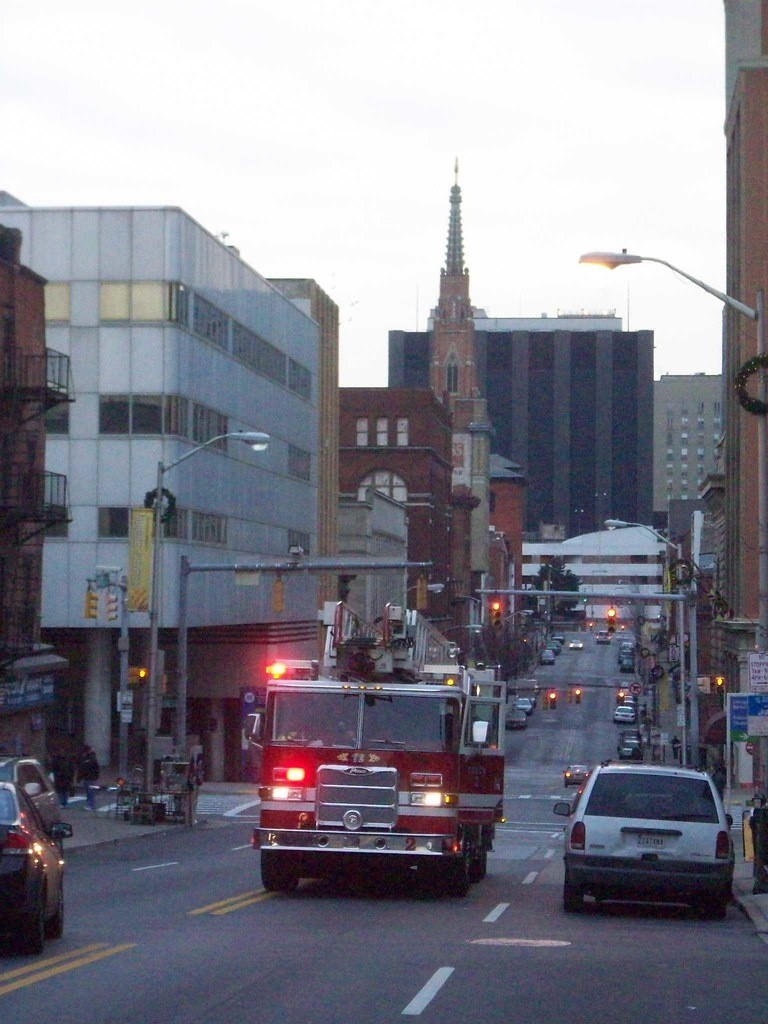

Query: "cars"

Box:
562,765,589,786
570,639,584,650
595,630,611,644
506,676,540,730
0,782,75,954
0,757,64,859
542,636,565,665
617,727,645,761
612,687,637,723
552,759,740,920
617,641,637,674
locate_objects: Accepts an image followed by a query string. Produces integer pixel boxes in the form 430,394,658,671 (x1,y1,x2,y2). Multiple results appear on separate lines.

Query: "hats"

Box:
80,744,90,753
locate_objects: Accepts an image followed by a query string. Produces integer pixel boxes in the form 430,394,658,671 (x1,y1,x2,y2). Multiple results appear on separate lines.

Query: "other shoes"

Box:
84,807,96,812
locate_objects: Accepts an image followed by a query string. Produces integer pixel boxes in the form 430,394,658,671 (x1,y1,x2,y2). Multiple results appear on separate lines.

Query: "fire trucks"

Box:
243,599,506,899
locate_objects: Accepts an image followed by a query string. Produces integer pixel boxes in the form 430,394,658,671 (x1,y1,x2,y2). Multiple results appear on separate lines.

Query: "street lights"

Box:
146,430,272,790
578,248,767,712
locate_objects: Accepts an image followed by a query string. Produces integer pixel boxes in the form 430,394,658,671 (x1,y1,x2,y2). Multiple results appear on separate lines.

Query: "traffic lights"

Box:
106,593,120,622
548,690,558,709
567,689,573,704
575,688,583,705
84,591,101,622
607,609,616,635
543,692,548,711
129,666,149,689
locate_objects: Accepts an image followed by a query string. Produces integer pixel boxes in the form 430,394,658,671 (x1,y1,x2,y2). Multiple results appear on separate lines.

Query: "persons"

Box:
671,734,768,852
51,740,101,811
311,709,353,746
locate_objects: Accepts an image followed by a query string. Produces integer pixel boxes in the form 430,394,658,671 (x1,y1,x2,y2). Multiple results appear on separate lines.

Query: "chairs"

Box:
670,790,700,817
601,791,627,810
